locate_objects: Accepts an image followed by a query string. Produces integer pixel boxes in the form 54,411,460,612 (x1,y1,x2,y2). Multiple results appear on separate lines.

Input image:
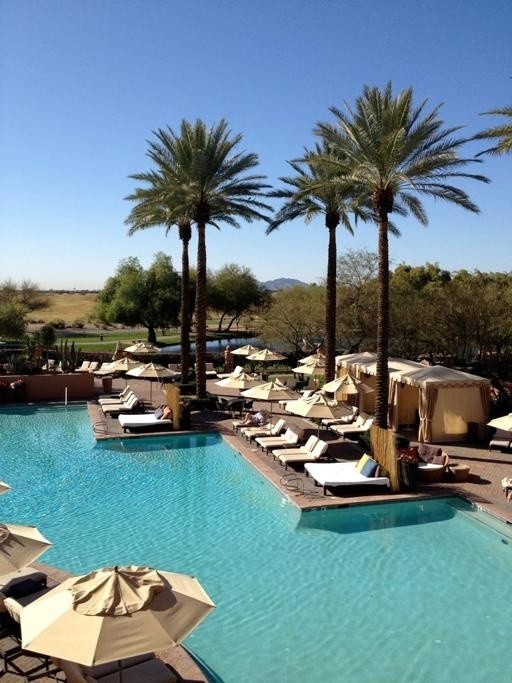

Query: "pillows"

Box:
155,404,172,419
417,445,446,464
355,452,389,477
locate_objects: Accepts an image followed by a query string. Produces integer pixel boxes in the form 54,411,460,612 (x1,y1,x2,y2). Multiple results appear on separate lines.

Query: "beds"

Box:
118,413,172,433
303,461,391,495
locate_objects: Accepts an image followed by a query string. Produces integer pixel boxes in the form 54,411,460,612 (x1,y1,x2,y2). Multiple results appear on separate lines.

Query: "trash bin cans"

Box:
467,421,479,446
102,376,112,395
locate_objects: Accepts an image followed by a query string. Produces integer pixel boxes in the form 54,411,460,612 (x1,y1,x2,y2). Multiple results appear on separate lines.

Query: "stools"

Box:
447,463,470,480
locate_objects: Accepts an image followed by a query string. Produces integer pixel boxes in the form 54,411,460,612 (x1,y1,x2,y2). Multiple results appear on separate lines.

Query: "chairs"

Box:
405,444,449,481
97,385,140,417
42,359,216,378
1,565,179,682
488,428,511,451
216,364,373,470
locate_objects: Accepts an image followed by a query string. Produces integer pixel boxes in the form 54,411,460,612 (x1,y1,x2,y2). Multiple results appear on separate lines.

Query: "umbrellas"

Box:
0,521,52,576
123,340,163,360
125,362,180,401
213,343,375,439
0,481,11,495
18,563,216,681
101,356,145,387
486,411,512,433
